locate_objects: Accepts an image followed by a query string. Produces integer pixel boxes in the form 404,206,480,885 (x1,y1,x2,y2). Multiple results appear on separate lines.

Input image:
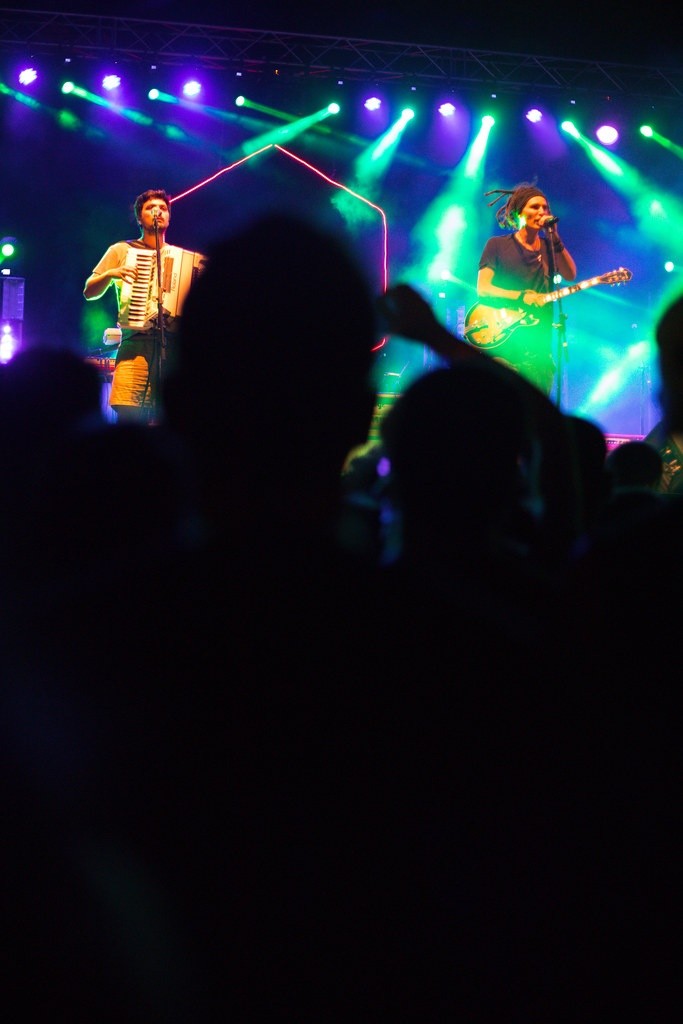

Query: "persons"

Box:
0,184,683,1024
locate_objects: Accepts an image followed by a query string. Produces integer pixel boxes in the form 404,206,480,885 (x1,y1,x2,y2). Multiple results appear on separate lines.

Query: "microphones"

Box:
152,210,161,221
542,217,559,227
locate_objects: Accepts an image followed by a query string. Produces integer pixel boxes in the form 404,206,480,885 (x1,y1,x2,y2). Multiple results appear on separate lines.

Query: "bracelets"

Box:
553,242,565,253
553,240,561,246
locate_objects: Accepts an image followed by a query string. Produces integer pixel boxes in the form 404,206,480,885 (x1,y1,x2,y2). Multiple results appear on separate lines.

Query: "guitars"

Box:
464,266,633,348
